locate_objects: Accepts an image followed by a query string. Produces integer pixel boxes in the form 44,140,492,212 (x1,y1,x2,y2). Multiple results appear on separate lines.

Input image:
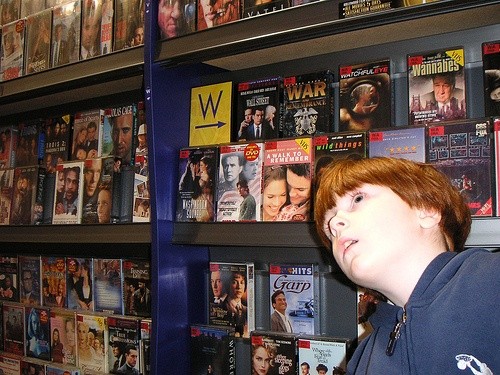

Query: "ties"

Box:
255,125,260,139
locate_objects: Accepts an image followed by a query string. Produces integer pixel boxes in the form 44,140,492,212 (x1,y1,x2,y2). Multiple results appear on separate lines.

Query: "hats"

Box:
137,124,148,135
109,330,128,348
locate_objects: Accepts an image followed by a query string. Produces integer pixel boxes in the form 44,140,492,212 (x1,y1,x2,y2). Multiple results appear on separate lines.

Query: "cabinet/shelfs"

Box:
0,0,500,375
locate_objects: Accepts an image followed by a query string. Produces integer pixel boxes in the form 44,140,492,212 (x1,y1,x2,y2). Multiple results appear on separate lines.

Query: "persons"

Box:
0,0,466,374
314,153,500,375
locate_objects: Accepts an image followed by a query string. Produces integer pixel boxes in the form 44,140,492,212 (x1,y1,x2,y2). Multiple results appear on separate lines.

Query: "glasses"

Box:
111,346,120,349
